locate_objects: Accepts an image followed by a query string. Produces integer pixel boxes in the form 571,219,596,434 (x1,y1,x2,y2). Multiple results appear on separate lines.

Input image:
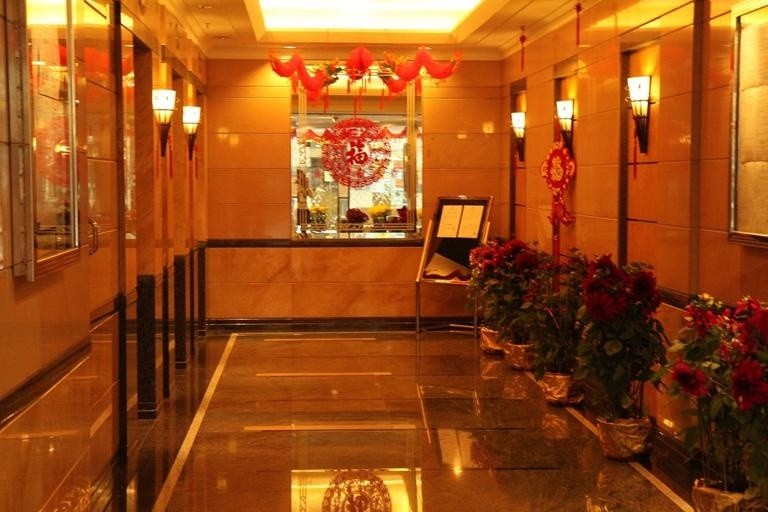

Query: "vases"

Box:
689,474,767,511
588,410,658,463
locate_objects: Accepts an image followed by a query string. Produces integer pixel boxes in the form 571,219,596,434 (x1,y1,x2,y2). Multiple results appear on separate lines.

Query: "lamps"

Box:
622,74,656,156
551,97,574,160
178,105,204,161
147,88,177,158
508,110,528,163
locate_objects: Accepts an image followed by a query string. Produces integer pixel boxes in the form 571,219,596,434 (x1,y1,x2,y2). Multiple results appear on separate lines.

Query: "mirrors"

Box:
287,63,428,241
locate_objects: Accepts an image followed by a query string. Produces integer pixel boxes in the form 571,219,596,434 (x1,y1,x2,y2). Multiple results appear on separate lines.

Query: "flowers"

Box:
305,204,409,225
645,282,768,491
462,237,674,423
